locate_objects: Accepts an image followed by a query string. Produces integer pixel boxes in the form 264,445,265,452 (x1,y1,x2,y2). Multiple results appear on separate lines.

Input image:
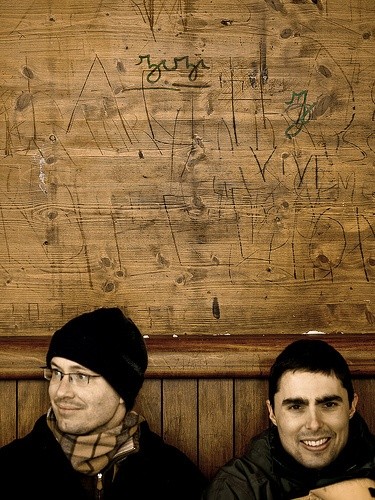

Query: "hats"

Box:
46,307,148,411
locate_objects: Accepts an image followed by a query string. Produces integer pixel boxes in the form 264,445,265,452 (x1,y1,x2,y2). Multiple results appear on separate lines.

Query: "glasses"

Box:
38,365,100,385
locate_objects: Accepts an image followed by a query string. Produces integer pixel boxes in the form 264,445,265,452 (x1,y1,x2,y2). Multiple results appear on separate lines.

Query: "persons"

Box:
205,339,375,500
1,305,208,500
